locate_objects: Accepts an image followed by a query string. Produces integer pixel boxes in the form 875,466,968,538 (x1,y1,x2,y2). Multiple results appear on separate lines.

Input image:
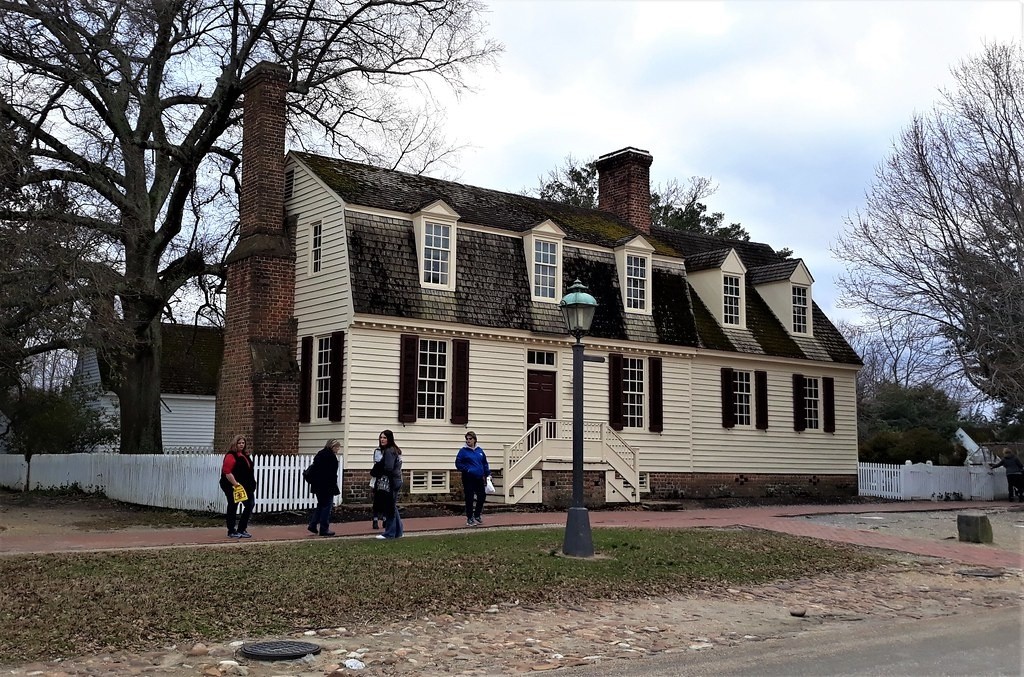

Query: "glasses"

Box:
466,438,473,440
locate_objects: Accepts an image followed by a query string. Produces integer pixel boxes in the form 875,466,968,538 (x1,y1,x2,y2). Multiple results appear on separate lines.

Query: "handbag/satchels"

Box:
303,464,313,484
485,476,496,494
233,484,248,503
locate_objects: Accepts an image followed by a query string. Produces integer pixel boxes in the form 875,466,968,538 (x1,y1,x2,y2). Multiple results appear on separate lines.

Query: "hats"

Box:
327,439,341,448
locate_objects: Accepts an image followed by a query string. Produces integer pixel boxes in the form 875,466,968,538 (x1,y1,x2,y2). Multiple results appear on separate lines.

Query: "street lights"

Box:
557,276,601,559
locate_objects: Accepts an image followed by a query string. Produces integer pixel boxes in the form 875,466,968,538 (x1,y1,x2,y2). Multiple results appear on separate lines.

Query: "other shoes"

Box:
382,521,386,528
373,521,379,529
467,518,477,526
376,535,386,539
473,516,483,525
320,530,335,536
307,526,318,534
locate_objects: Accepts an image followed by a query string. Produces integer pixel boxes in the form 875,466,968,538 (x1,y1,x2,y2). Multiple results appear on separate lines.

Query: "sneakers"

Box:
228,532,242,538
238,531,252,538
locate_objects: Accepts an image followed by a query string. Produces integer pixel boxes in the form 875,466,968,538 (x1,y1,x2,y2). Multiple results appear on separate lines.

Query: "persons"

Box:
302,438,341,537
219,435,257,539
454,431,493,526
369,429,404,540
